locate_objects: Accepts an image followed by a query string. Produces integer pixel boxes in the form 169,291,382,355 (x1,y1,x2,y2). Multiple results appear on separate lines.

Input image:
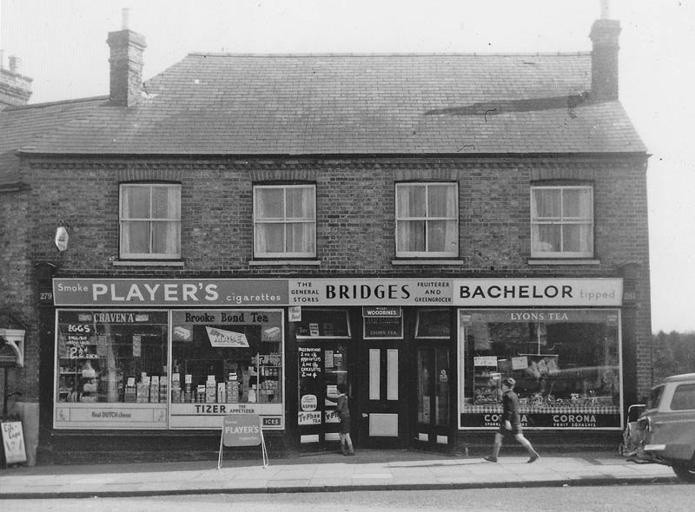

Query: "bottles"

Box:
260,367,278,390
190,390,205,403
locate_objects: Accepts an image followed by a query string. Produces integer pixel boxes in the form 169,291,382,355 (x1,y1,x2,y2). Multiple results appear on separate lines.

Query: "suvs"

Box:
614,372,695,481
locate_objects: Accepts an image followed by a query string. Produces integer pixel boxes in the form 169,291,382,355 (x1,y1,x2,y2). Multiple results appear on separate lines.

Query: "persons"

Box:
482,376,541,463
325,385,356,455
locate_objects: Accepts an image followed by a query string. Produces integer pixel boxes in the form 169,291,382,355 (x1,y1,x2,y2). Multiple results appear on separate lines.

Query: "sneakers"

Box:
484,456,498,462
527,453,539,463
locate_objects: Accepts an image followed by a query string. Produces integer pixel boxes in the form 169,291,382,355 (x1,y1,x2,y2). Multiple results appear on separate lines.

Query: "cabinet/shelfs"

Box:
466,328,618,406
58,330,281,404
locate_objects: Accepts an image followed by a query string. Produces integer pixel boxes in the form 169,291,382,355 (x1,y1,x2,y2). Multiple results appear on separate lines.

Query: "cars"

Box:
543,364,619,405
